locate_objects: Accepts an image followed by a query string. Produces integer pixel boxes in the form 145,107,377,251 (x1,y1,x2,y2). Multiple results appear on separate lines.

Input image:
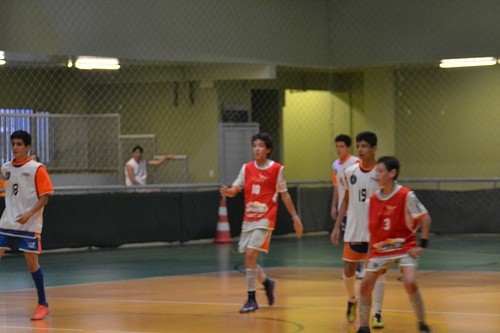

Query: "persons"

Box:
220,133,303,312
0,130,54,320
332,131,430,333
124,146,171,185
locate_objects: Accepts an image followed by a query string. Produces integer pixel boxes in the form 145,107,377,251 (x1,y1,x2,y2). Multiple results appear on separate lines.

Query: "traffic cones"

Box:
212,198,234,244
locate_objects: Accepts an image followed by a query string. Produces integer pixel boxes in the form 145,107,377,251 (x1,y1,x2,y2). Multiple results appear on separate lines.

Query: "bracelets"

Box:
292,216,299,223
419,239,427,248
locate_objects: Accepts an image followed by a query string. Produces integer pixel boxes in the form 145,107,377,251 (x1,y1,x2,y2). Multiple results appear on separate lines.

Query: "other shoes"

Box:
263,279,276,306
239,298,259,313
355,326,370,333
372,313,384,328
418,321,429,333
346,296,358,322
30,304,50,320
